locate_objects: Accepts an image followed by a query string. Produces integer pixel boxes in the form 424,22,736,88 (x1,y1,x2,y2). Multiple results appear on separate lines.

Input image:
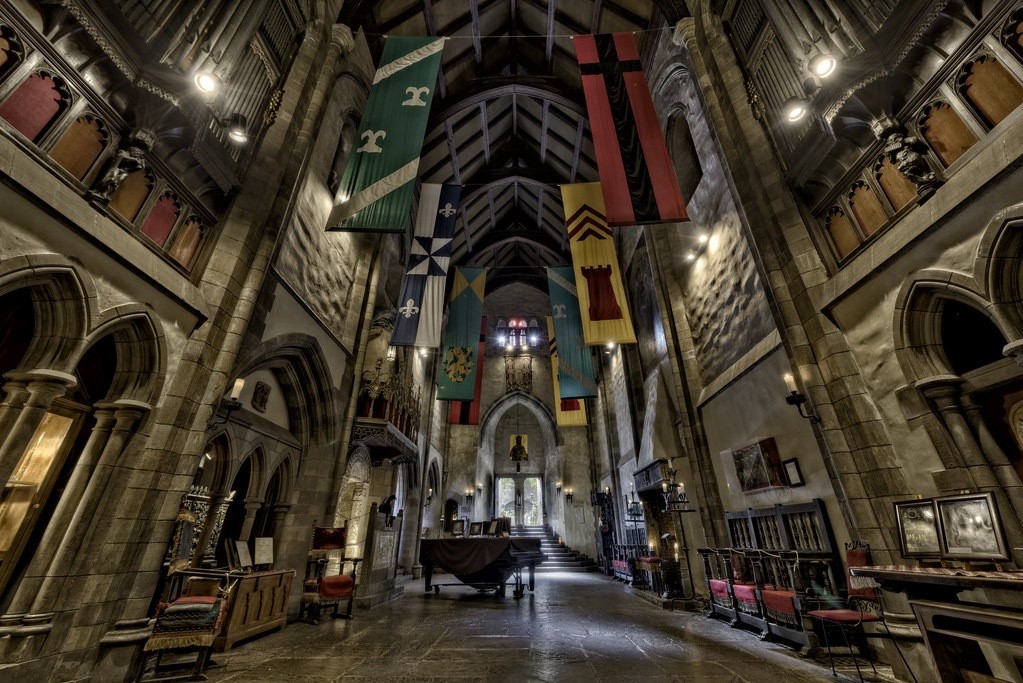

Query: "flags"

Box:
386,183,461,349
560,182,637,343
546,316,587,427
433,266,487,400
573,32,689,225
326,36,444,231
548,265,600,399
447,317,486,426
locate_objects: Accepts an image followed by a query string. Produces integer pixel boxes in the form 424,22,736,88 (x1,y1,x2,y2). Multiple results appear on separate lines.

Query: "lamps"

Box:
206,451,215,460
784,374,820,425
424,489,432,508
807,54,837,78
783,97,809,122
209,379,245,431
221,113,246,141
605,487,613,503
662,459,711,612
803,78,822,95
466,483,482,504
628,482,642,529
556,483,574,505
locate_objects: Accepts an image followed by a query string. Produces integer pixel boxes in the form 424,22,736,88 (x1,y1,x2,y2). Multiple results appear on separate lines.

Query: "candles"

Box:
675,554,679,562
650,544,654,551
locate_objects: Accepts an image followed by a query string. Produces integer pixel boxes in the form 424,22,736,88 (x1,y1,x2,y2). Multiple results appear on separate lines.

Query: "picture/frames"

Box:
452,517,511,534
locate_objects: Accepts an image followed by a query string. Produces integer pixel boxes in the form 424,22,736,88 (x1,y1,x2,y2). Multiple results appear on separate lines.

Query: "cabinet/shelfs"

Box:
849,565,1023,683
182,565,297,652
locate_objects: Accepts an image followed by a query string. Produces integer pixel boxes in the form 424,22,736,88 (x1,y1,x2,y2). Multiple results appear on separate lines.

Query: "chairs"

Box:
137,571,232,683
696,498,878,659
805,539,919,683
610,528,650,589
296,519,364,625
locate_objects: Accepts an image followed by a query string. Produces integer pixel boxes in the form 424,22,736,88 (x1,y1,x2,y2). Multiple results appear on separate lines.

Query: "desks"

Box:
638,555,663,595
